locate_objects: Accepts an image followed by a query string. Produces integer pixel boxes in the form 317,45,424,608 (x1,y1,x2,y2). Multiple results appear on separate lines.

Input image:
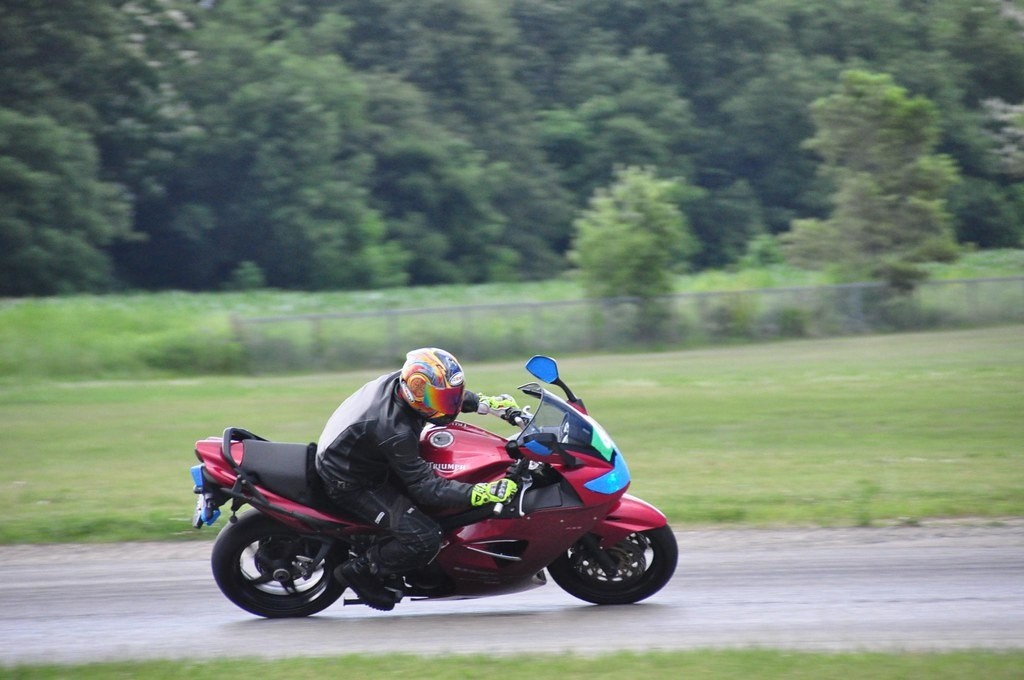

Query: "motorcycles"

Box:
190,354,678,619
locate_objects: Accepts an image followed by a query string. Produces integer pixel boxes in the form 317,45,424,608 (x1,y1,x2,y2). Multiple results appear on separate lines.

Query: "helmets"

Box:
399,348,465,425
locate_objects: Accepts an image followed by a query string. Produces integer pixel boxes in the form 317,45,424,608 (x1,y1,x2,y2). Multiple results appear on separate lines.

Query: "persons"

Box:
314,347,521,611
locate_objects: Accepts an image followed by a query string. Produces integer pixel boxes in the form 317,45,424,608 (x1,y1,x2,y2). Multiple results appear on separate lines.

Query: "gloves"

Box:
470,479,519,508
477,394,522,420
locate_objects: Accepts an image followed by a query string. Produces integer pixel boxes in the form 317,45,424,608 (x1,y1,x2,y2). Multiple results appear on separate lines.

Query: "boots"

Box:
333,544,394,611
